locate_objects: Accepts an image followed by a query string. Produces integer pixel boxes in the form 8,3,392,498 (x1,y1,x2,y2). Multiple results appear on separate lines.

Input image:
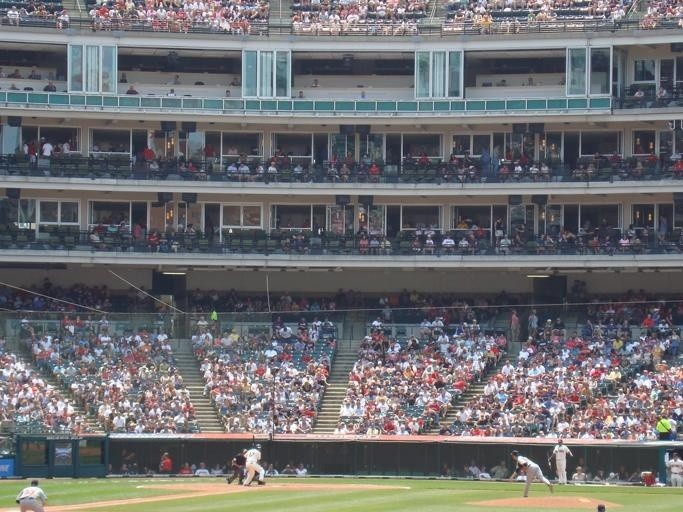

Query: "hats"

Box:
510,450,519,455
162,453,168,457
0,315,683,439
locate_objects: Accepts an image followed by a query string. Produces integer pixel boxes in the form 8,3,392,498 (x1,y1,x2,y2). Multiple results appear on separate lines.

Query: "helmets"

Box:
256,444,261,449
31,480,38,487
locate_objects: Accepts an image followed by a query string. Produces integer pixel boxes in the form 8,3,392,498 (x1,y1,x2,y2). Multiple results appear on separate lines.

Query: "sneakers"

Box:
548,484,554,493
226,478,265,486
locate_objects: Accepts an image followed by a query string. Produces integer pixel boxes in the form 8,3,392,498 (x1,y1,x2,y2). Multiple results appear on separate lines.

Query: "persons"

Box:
508,450,552,497
15,479,48,512
